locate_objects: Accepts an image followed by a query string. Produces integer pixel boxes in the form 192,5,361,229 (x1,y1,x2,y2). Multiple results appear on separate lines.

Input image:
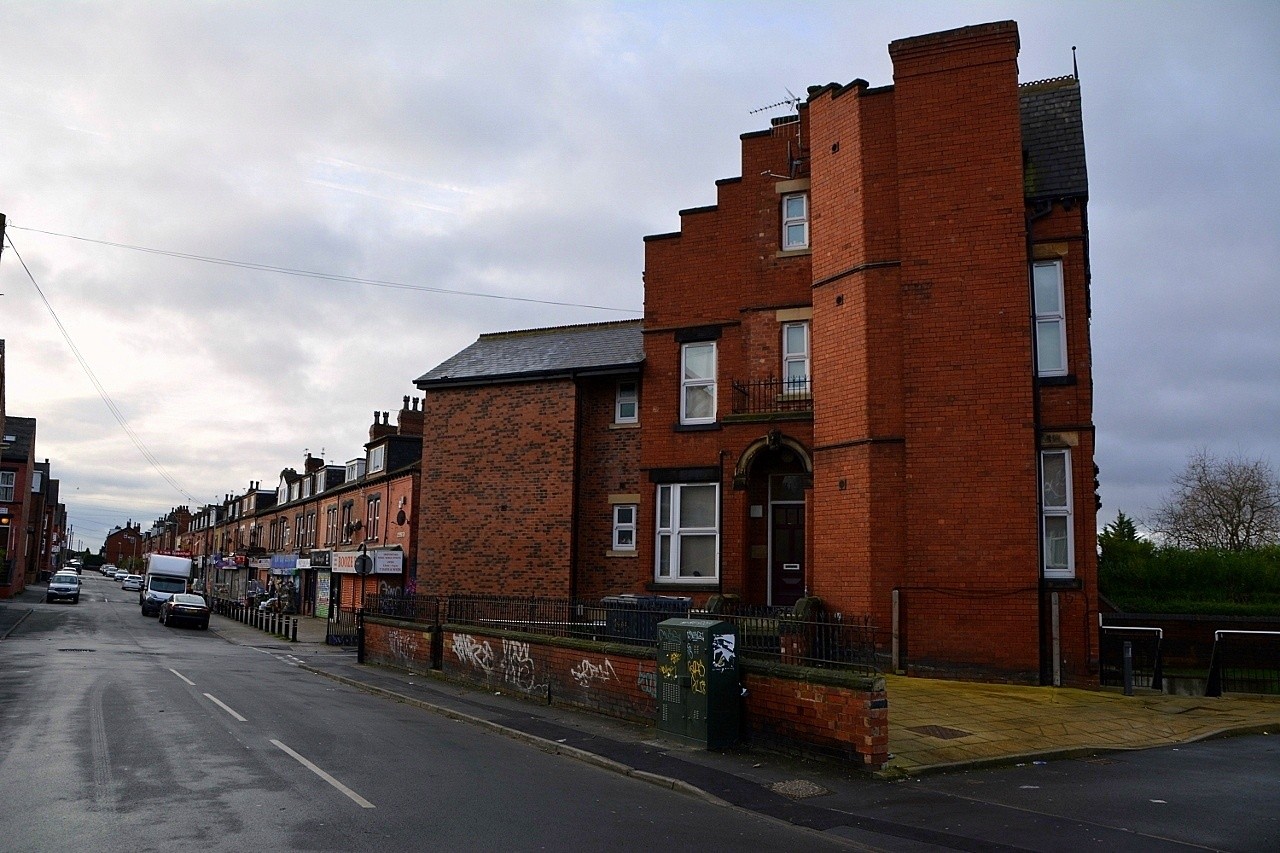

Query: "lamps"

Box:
766,427,783,450
732,474,748,491
397,510,410,526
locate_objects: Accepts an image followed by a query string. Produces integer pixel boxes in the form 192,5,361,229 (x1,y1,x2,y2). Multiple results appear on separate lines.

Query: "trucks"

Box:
139,553,193,616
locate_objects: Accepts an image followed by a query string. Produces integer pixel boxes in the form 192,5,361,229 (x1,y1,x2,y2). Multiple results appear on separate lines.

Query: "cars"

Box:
46,574,81,604
122,575,143,591
100,564,115,576
107,567,119,577
53,558,83,582
160,592,211,630
114,570,130,582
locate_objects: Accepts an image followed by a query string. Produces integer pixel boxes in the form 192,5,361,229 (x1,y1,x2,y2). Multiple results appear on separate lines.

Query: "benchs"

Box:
730,605,796,650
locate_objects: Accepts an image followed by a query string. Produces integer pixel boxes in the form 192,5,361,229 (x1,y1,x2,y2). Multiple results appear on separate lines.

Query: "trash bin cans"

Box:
602,593,692,647
